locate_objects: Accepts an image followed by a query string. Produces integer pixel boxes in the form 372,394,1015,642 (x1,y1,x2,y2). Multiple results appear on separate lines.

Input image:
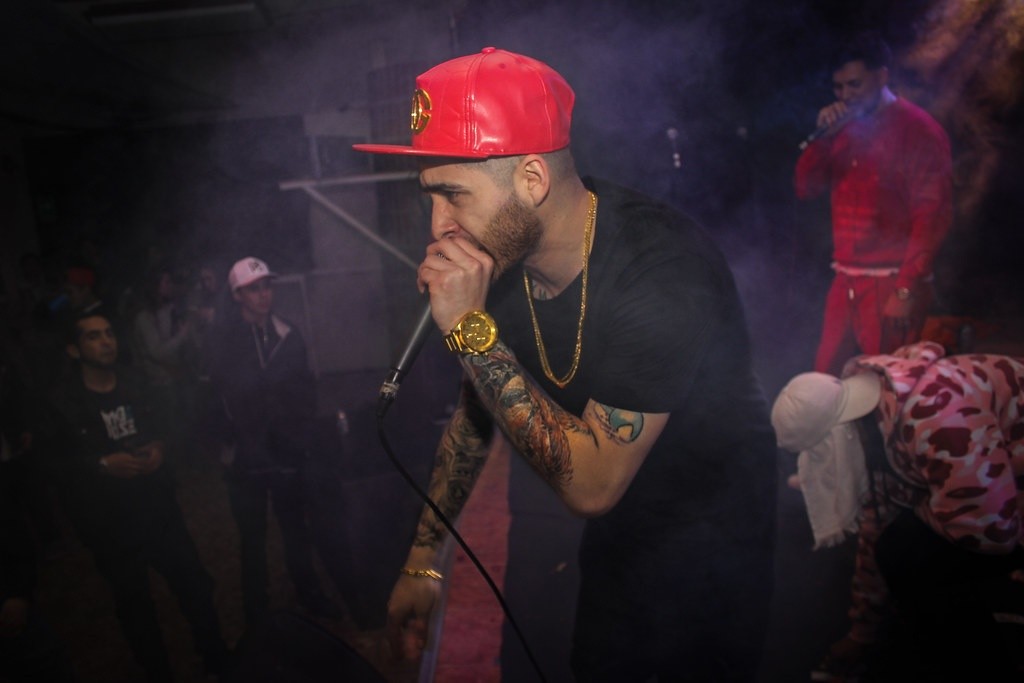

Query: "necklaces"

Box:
523,191,597,389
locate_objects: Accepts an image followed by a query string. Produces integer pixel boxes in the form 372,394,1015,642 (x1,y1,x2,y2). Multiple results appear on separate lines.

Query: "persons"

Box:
352,45,779,683
788,31,952,491
47,255,341,682
767,340,1024,683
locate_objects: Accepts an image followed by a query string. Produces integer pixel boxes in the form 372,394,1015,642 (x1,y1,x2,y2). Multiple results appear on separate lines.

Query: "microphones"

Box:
798,122,829,150
376,252,449,414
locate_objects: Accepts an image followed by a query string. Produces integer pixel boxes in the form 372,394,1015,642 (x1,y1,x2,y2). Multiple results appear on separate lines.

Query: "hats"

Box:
351,46,575,159
770,371,882,453
227,256,277,294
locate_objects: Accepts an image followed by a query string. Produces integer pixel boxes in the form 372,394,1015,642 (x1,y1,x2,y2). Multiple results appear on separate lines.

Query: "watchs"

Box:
441,310,498,356
893,286,919,301
98,458,109,476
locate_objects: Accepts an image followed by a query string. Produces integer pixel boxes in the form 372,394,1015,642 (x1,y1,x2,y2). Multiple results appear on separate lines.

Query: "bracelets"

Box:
401,566,448,582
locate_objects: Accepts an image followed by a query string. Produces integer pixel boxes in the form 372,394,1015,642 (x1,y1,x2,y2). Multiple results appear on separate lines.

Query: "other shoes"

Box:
787,471,802,492
